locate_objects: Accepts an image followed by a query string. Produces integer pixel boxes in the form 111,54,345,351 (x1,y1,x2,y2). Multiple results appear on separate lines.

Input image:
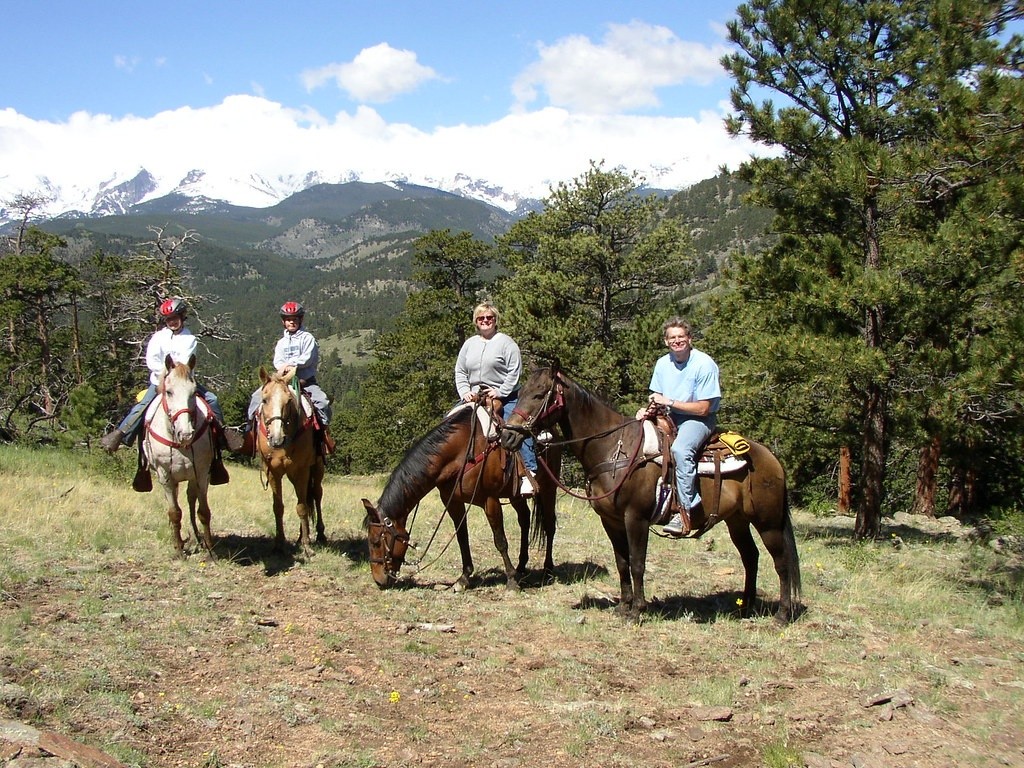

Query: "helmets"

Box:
159,299,186,318
279,302,305,323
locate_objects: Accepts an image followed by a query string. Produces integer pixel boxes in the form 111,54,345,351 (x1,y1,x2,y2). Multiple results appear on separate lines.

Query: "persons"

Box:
442,303,540,496
635,316,722,535
102,298,243,451
241,301,336,455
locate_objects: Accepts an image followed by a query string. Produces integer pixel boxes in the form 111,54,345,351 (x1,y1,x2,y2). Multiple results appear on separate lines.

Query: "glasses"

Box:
478,316,494,321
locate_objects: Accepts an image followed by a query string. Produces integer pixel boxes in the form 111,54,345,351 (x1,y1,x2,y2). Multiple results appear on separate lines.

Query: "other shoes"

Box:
521,471,536,495
663,513,690,535
101,430,126,451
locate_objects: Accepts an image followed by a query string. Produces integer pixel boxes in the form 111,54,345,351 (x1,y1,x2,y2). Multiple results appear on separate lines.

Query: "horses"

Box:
140,354,218,561
500,358,803,626
247,365,333,558
359,398,565,597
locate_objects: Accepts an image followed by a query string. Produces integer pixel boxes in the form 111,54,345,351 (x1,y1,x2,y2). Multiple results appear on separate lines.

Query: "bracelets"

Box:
669,399,675,406
